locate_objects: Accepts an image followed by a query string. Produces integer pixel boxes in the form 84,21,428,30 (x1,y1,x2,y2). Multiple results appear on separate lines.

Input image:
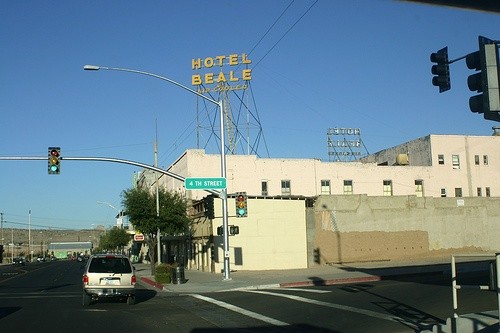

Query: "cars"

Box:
72,256,89,262
36,255,56,263
11,258,23,264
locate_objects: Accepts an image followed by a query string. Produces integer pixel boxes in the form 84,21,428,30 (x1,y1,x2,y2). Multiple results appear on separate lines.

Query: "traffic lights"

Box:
430,47,450,94
48,146,62,175
230,227,240,234
217,227,224,235
0,245,5,263
464,36,500,113
236,192,247,217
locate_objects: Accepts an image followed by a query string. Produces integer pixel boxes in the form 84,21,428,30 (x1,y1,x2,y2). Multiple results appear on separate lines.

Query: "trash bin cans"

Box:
172,266,185,284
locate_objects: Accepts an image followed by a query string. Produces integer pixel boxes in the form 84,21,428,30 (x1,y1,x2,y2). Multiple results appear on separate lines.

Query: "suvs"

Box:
82,251,135,307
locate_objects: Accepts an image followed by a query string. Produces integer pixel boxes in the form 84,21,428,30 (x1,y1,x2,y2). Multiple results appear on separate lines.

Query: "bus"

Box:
48,242,90,257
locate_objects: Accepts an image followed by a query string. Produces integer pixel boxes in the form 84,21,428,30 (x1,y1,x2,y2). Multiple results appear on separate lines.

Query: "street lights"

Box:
94,201,123,254
91,224,107,252
84,66,230,280
